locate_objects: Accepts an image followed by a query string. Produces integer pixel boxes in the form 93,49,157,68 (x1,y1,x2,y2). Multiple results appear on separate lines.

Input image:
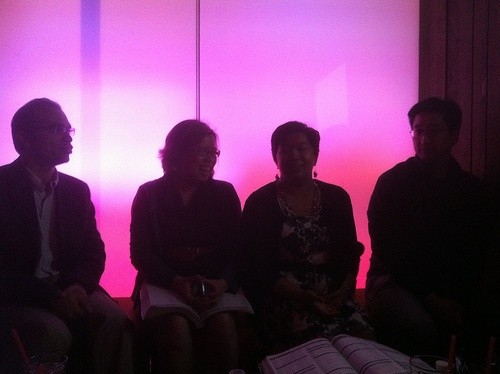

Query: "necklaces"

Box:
276,176,332,258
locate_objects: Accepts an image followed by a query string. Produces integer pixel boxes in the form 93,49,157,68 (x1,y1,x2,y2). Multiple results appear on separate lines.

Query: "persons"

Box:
238,121,376,344
129,119,243,374
365,96,500,363
0,97,134,374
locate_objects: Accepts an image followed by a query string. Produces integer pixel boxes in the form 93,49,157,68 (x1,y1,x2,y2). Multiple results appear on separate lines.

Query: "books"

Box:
139,281,256,330
257,334,438,373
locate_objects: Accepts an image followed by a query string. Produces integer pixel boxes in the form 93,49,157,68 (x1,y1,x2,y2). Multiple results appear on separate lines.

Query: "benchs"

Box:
109,288,376,368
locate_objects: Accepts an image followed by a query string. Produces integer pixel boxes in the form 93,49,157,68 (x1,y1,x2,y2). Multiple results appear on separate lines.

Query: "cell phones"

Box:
201,281,205,297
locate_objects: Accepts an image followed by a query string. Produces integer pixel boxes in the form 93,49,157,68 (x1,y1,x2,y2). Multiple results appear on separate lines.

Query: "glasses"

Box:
192,146,220,159
409,127,451,139
36,125,72,136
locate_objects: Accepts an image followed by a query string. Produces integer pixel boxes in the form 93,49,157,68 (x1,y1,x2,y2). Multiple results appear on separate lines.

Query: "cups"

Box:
17,352,69,374
468,358,500,374
409,354,457,374
